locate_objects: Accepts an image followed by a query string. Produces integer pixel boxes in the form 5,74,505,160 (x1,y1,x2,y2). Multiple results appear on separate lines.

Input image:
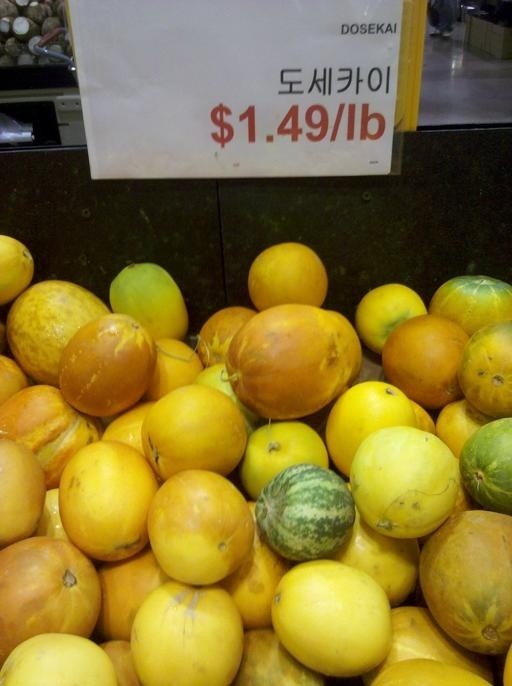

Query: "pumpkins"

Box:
0,234,512,686
